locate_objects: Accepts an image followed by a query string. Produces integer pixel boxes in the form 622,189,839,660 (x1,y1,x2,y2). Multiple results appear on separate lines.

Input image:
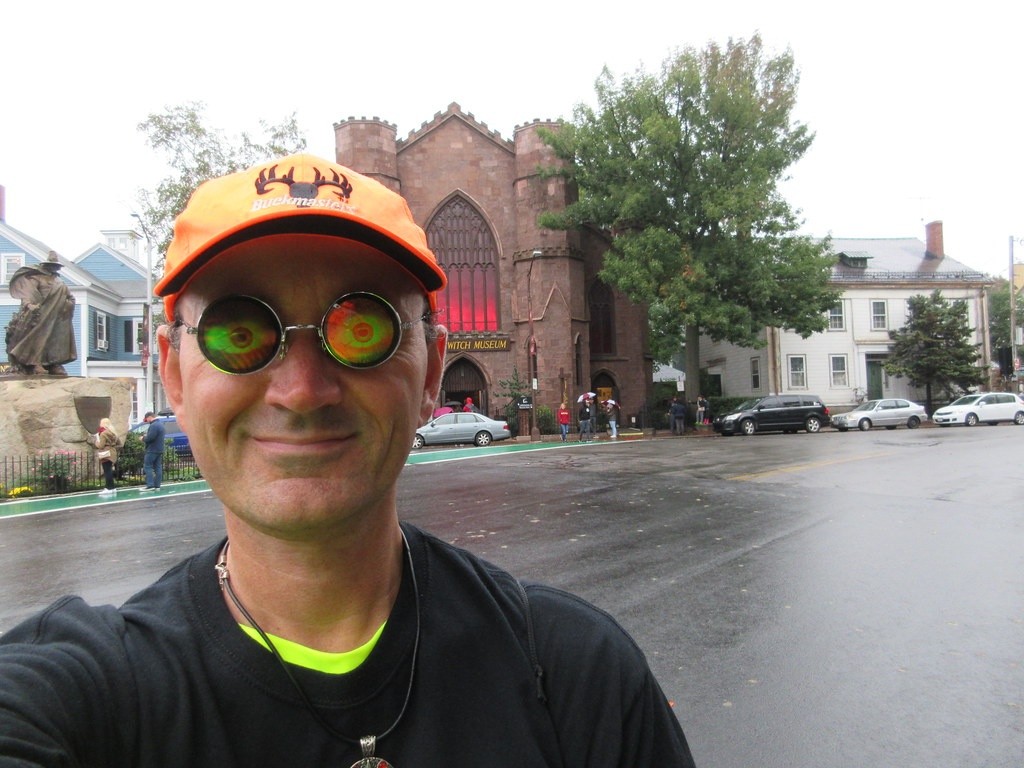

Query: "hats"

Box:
153,153,448,325
144,412,153,422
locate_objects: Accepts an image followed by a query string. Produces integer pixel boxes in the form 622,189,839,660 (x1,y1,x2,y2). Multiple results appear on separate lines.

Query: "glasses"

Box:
177,291,426,376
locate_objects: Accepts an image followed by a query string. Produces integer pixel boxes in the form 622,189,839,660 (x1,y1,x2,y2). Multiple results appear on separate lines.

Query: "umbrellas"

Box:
603,399,620,409
433,407,452,417
577,392,596,403
444,401,462,406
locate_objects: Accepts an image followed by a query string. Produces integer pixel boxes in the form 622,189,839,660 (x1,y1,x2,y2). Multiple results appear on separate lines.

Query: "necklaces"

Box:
214,522,421,768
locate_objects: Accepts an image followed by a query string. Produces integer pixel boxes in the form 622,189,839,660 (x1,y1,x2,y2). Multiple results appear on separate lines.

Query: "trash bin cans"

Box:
627,413,637,429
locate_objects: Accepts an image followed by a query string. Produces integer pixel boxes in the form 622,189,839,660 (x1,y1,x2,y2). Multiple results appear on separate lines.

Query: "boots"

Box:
704,418,709,425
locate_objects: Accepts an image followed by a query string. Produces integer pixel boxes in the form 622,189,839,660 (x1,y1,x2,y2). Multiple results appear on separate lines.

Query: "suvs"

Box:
712,394,832,437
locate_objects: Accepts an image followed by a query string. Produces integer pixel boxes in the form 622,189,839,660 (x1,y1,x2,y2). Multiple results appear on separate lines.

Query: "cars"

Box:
832,399,928,431
407,412,511,448
123,416,193,457
931,392,1024,426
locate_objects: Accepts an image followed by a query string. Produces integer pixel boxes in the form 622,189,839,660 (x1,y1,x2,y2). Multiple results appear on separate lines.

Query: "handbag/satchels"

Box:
98,450,110,460
698,407,705,411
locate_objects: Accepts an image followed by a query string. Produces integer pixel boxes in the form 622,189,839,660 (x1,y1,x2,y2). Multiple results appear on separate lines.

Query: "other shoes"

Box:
139,487,160,491
695,422,703,425
610,435,617,438
98,488,116,493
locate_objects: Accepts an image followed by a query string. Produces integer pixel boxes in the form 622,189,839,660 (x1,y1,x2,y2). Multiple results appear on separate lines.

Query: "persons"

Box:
578,400,593,444
138,411,165,491
589,400,596,437
666,395,687,436
95,418,121,495
6,249,78,376
462,397,473,412
606,404,618,439
0,152,693,768
688,395,710,425
558,403,570,443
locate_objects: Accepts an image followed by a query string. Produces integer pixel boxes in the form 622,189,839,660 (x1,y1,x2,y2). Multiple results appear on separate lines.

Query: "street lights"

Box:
530,249,542,440
131,212,154,414
